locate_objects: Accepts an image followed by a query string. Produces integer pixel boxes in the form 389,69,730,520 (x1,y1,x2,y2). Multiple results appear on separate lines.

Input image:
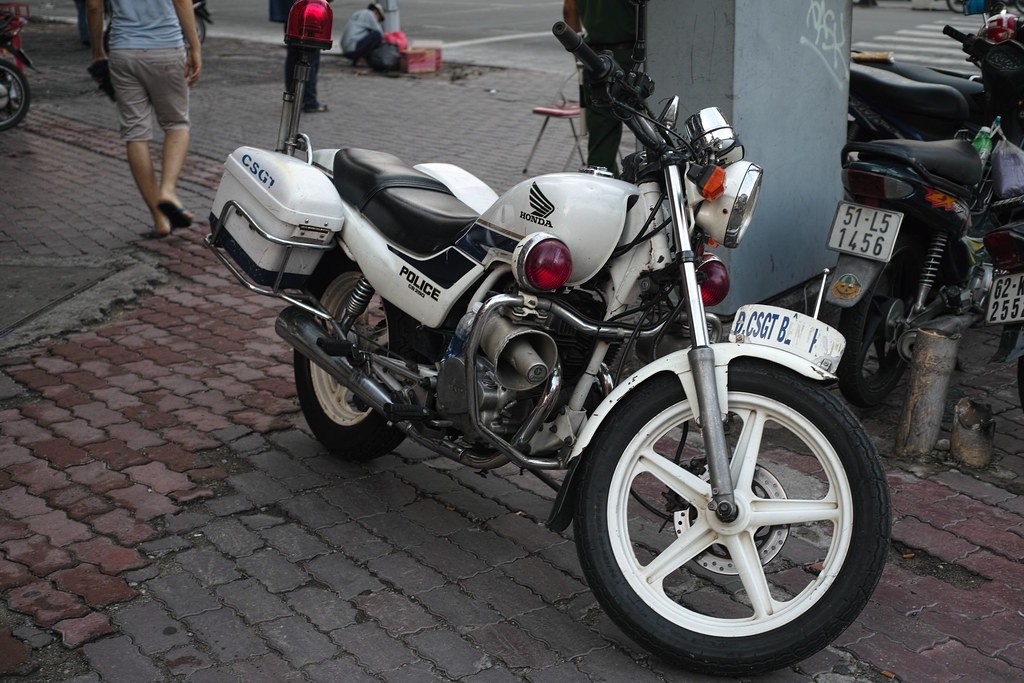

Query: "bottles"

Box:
971,125,993,189
439,301,484,368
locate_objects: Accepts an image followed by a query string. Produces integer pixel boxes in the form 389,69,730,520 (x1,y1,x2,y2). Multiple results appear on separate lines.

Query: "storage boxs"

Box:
401,46,442,73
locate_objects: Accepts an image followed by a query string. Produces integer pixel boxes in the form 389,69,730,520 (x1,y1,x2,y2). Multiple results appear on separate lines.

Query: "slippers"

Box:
158,200,191,227
153,221,171,237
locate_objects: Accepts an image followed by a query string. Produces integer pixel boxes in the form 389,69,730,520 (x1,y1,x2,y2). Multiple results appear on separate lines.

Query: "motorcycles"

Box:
820,9,1023,410
203,0,896,677
0,8,40,134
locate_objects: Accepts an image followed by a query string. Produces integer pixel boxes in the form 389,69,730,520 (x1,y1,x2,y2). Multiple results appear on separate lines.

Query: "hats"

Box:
369,3,385,22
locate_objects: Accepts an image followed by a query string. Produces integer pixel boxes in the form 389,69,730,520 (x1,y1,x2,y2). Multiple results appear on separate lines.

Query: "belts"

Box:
587,40,636,51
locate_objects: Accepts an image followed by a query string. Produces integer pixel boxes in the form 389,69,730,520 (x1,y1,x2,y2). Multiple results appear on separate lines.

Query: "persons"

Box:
563,0,645,176
268,0,328,112
341,3,385,67
85,0,201,237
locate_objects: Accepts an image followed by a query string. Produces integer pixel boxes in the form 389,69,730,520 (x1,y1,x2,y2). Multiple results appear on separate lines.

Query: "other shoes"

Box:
355,63,368,67
305,105,327,111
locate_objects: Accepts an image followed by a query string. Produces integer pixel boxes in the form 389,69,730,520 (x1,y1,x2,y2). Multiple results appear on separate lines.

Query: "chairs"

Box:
521,67,623,175
